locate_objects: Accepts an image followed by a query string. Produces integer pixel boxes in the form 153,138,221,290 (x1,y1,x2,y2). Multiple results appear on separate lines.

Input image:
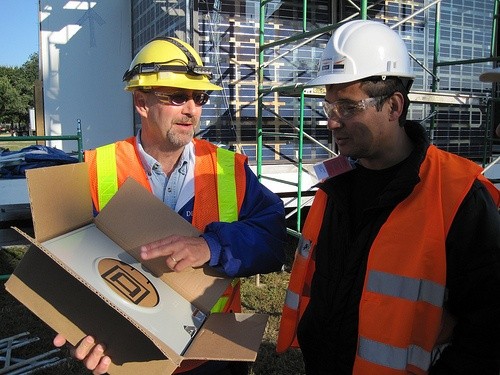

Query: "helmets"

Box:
121,36,223,92
304,19,416,86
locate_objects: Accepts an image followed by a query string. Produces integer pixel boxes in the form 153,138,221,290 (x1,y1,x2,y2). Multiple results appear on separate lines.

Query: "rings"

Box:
170,255,178,264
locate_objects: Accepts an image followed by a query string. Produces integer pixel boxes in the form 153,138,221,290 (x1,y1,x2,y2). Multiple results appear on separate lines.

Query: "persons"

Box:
51,35,287,375
276,20,500,375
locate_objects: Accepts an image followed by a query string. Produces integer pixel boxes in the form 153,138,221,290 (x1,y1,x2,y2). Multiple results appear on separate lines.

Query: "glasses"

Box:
140,90,210,106
322,96,387,119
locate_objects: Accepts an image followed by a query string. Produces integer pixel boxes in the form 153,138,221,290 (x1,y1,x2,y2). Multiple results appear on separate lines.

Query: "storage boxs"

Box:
4,161,270,374
